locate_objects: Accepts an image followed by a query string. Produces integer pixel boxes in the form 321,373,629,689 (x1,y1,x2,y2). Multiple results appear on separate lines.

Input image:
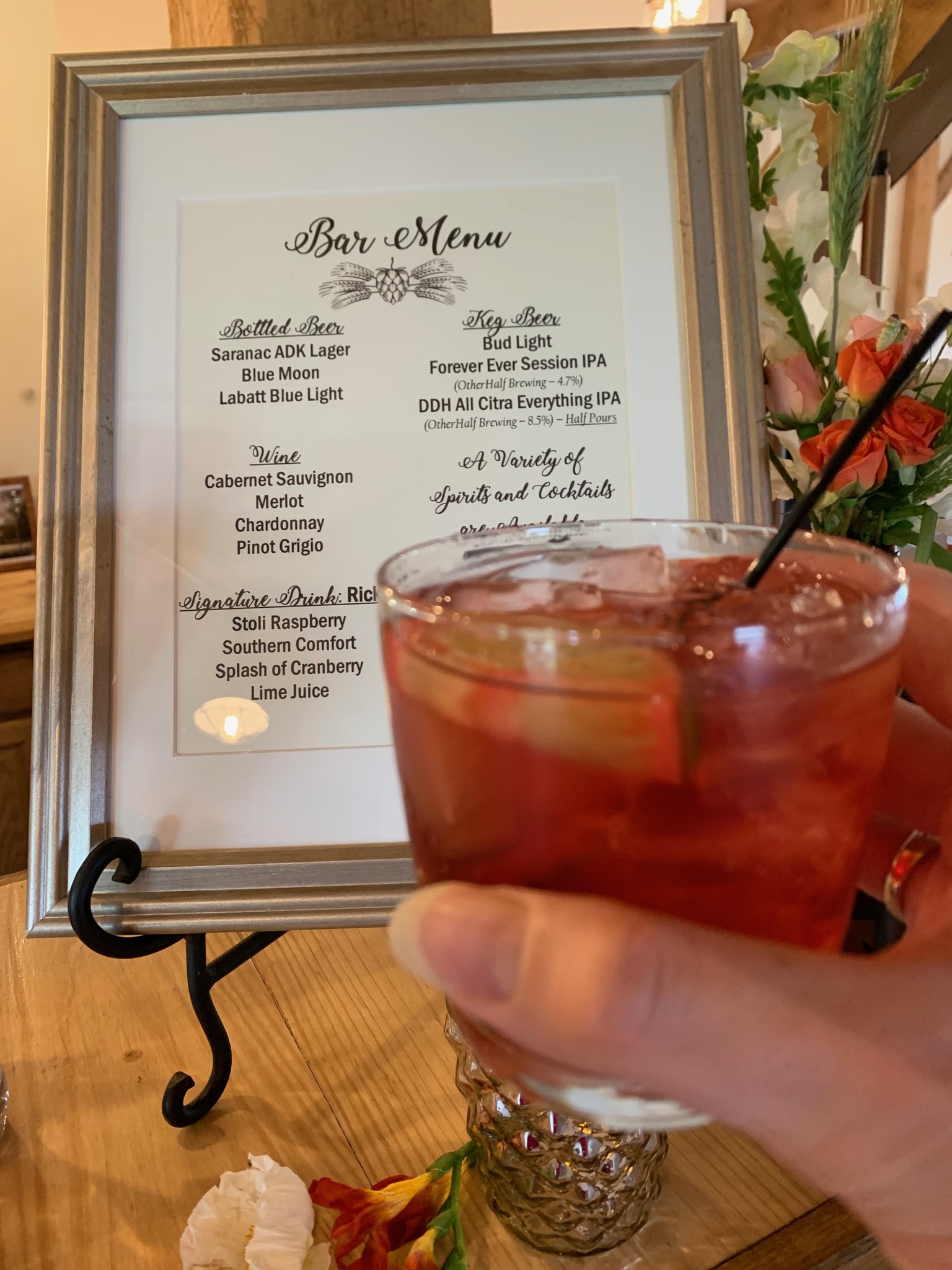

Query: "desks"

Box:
0,869,908,1270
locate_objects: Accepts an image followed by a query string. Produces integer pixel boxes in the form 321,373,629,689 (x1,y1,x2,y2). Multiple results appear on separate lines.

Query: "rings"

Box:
880,828,944,923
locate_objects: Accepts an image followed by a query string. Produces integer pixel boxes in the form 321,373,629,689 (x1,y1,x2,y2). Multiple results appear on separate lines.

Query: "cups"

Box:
445,1013,671,1256
377,521,908,1130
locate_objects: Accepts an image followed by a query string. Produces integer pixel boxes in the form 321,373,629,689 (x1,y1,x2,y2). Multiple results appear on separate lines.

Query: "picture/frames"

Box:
24,23,774,935
0,475,44,572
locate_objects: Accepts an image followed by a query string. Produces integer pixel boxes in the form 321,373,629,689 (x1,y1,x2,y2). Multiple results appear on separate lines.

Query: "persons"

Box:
384,522,952,1270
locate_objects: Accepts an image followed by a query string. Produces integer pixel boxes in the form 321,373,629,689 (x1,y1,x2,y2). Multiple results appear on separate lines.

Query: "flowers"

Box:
178,1154,471,1270
730,0,952,572
